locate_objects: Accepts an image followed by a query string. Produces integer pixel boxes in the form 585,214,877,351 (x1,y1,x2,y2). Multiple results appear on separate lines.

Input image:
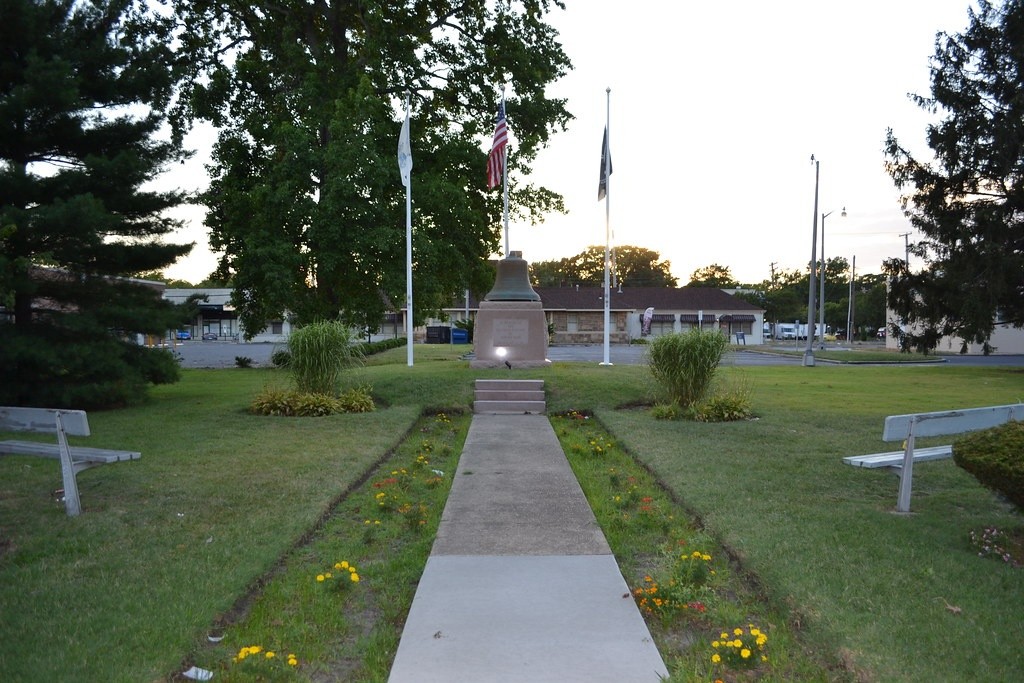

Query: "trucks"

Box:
777,325,796,340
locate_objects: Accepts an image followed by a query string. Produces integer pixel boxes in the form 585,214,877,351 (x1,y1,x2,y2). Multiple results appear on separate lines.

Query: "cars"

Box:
835,330,846,337
175,330,191,340
203,333,217,340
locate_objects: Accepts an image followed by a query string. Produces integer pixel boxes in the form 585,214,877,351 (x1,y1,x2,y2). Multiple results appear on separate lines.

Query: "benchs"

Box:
841,404,1024,510
0,407,142,516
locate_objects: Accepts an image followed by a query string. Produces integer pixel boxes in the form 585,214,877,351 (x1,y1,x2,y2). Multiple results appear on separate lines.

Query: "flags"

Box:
598,126,613,202
487,99,507,189
397,115,412,184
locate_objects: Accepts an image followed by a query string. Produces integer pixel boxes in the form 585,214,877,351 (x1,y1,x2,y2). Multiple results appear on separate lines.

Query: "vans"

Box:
763,327,771,338
876,327,886,338
